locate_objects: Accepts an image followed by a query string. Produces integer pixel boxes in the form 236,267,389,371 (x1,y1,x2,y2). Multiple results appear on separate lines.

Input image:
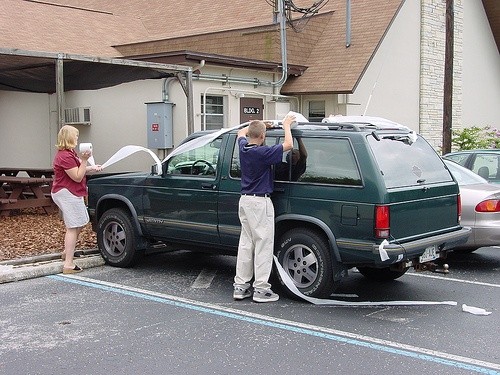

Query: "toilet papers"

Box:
321,115,418,143
78,111,311,175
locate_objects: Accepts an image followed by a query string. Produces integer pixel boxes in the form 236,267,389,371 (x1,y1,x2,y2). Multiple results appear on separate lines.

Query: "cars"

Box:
441,148,500,182
439,156,500,253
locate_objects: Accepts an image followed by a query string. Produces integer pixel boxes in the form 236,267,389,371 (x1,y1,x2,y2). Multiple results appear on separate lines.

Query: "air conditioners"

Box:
64,107,91,125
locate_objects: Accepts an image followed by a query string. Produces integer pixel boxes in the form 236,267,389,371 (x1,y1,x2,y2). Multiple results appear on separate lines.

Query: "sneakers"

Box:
253,290,280,303
233,289,252,299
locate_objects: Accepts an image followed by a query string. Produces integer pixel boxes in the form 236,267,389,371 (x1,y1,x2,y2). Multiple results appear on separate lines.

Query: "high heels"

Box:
61,250,81,259
63,263,83,274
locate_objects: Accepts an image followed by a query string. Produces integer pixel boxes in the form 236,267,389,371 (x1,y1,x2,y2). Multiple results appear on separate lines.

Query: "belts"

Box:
242,193,272,197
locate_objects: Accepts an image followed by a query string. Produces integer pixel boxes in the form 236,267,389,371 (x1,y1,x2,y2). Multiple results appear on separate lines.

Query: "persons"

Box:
232,113,296,303
51,125,103,273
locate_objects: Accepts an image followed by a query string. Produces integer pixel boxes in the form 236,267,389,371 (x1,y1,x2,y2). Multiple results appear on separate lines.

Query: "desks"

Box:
0,176,53,217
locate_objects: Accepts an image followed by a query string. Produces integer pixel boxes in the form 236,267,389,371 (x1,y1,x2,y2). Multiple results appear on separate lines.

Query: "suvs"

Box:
86,114,473,304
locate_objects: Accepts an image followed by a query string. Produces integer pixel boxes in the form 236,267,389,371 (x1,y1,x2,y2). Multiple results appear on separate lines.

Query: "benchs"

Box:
6,190,54,216
